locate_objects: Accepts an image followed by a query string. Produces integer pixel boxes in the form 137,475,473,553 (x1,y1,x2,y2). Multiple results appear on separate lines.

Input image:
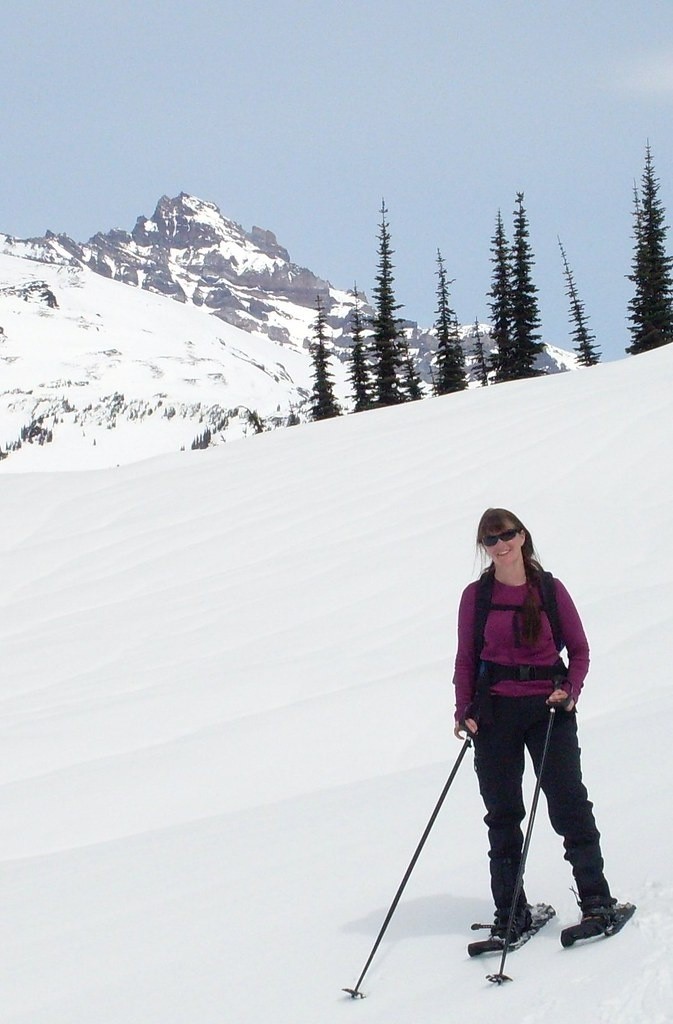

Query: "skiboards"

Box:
468,903,636,958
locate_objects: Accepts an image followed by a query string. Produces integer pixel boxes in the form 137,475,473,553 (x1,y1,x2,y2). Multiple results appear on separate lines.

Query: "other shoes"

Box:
583,898,616,929
493,909,530,942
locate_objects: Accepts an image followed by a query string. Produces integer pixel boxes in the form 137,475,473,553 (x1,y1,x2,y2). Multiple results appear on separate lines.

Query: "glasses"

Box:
483,527,521,546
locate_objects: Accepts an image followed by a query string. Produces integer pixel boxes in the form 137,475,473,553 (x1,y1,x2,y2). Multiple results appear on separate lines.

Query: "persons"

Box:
452,507,616,944
